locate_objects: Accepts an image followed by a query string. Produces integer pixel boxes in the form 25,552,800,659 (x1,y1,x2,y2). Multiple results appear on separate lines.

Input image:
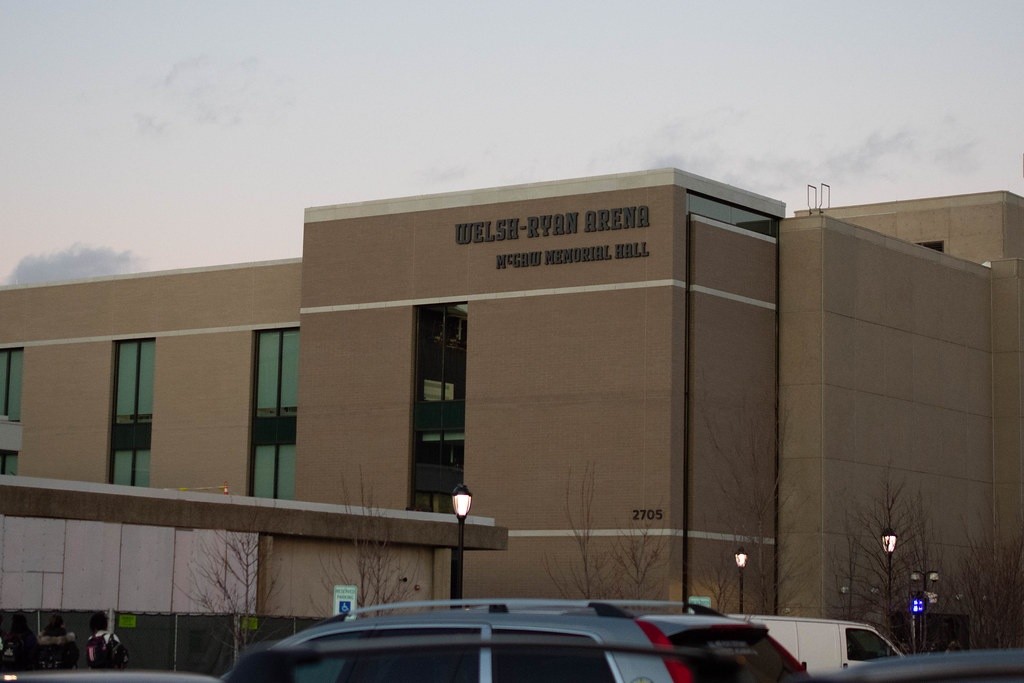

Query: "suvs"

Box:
223,597,810,683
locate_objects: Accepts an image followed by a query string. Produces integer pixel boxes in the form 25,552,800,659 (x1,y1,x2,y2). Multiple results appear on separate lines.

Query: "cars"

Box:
809,644,1024,683
0,668,221,683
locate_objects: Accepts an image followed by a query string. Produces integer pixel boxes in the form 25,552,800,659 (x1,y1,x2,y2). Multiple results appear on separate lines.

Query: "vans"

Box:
719,611,906,672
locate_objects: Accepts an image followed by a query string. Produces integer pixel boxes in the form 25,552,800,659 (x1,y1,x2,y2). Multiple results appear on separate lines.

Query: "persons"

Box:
0,614,80,672
946,640,961,656
88,612,120,669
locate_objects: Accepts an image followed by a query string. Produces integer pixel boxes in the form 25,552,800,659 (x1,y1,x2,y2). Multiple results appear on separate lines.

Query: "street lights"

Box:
880,527,898,640
909,571,941,649
451,483,472,599
734,547,749,613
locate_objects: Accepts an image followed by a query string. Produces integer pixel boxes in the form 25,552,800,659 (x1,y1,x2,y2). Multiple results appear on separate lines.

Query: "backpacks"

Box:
1,633,29,670
86,632,108,668
106,633,129,669
